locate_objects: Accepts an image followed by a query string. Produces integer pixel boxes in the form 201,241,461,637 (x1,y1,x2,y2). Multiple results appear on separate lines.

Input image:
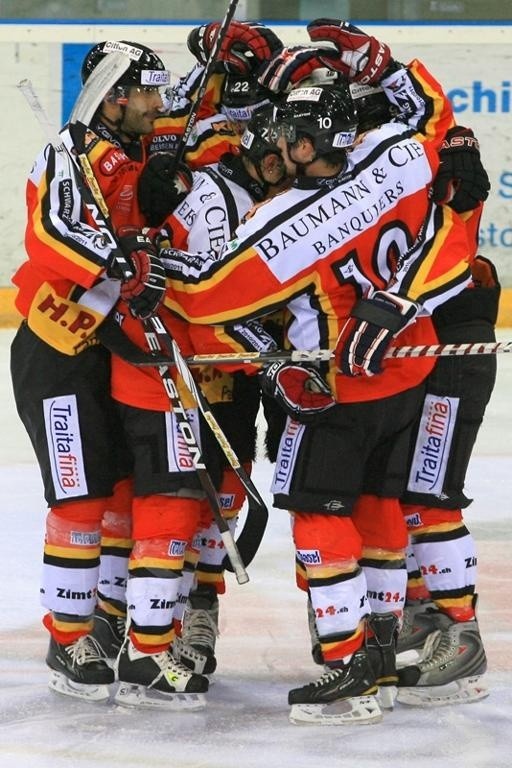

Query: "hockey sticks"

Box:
15,50,268,586
66,48,251,587
17,79,268,579
170,1,241,157
93,317,512,368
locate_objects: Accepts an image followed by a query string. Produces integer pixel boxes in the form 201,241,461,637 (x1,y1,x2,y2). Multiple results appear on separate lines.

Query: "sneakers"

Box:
45,634,117,685
309,590,487,686
288,649,377,704
91,596,218,676
118,638,211,695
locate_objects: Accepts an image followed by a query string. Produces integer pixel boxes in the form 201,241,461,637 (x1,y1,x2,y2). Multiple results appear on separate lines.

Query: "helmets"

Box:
81,39,168,105
220,67,387,165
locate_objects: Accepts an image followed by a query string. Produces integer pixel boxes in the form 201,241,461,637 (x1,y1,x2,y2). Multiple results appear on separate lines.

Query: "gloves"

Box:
118,230,167,320
431,125,489,213
265,356,337,419
333,287,422,380
186,16,392,97
